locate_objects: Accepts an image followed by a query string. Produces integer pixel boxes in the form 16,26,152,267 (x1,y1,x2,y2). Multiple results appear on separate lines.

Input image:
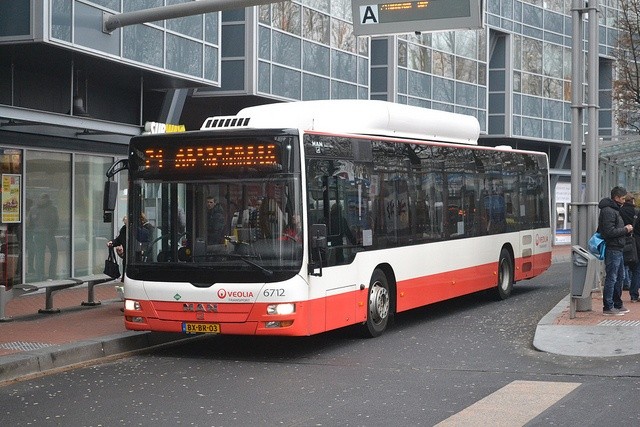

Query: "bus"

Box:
104,98,554,340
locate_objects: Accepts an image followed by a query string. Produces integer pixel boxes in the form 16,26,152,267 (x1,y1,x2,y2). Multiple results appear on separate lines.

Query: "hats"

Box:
625,191,635,201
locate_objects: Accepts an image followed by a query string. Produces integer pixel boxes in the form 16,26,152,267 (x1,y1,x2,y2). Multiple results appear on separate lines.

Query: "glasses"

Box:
291,212,299,217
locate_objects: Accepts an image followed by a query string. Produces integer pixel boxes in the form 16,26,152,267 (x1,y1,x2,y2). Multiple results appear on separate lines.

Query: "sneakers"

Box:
630,295,640,302
603,307,624,316
623,285,630,290
617,307,630,313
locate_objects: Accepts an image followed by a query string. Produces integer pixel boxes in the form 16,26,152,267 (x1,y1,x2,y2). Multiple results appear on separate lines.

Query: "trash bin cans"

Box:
571,245,597,298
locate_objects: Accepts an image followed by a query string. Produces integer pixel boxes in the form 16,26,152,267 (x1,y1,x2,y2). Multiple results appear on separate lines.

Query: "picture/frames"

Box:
1,174,22,224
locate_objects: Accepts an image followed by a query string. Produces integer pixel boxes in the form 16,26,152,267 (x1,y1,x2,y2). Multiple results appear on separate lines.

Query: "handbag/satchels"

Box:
588,232,606,260
622,237,639,265
103,260,121,279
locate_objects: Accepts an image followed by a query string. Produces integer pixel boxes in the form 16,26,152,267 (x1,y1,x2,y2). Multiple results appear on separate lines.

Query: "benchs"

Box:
67,273,113,305
13,279,82,314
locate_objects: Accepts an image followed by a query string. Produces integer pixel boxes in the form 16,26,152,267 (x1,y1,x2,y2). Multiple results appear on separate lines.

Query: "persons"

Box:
630,210,639,303
30,193,60,282
249,200,265,241
320,203,354,265
137,210,153,264
167,194,185,262
205,195,226,262
595,186,634,316
620,193,639,291
6,196,34,275
277,210,302,243
105,216,142,313
